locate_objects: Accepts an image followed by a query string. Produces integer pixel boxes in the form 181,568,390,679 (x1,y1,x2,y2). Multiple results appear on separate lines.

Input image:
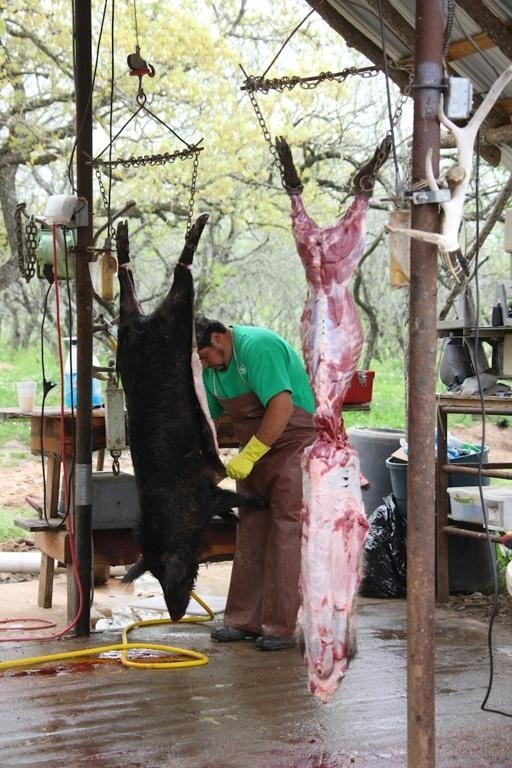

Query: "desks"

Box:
437,392,512,601
0,408,236,632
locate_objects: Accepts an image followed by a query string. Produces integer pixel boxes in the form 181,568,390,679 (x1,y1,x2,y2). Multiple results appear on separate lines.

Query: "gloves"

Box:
225,434,271,480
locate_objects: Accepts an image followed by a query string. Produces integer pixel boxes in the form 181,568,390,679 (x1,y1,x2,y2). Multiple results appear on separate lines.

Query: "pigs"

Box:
111,213,280,627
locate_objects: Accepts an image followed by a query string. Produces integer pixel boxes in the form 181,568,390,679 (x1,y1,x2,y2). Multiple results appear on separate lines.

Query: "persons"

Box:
194,317,317,651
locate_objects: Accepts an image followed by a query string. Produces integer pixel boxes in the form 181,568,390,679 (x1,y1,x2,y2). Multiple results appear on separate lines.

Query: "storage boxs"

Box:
447,486,512,531
90,471,139,530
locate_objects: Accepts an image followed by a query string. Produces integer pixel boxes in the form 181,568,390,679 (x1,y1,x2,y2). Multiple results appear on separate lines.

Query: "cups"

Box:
16,381,38,414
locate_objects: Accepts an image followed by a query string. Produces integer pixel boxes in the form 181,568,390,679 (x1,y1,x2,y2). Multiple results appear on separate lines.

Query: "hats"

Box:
195,311,210,349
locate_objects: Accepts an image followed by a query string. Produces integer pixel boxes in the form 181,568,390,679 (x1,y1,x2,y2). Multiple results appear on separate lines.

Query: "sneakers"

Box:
256,634,297,649
211,625,263,641
